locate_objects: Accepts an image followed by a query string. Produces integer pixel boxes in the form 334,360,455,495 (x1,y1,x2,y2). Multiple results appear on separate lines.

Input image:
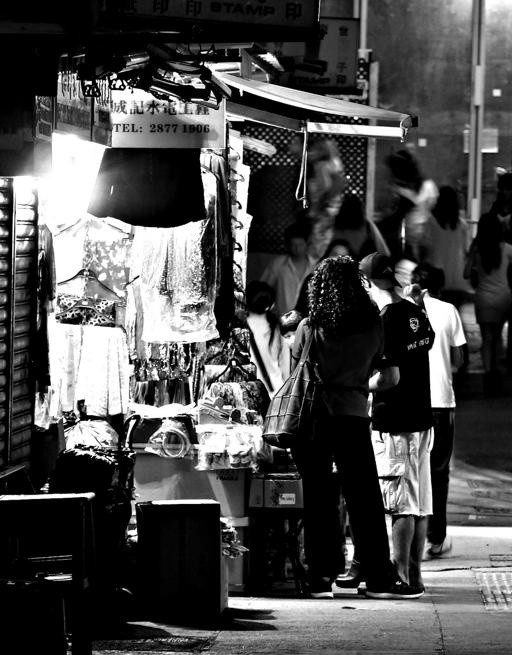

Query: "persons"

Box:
284,253,422,599
356,250,439,590
245,130,512,407
396,262,472,562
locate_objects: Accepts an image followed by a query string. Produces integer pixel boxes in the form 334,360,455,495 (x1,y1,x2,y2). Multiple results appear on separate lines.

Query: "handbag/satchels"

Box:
261,360,334,448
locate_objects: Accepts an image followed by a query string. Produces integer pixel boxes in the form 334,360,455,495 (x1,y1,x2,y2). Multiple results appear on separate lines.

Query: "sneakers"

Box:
428,540,451,556
303,571,426,599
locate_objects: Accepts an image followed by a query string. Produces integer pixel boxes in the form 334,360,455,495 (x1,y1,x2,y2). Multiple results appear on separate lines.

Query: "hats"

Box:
360,251,401,290
